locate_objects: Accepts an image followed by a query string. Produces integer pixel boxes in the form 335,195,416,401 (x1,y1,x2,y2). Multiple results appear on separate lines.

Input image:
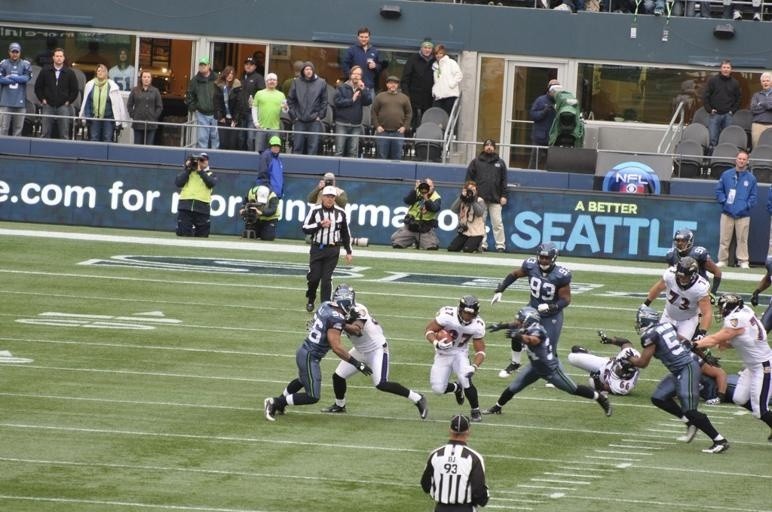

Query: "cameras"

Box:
325,179,333,186
417,179,430,193
467,189,474,198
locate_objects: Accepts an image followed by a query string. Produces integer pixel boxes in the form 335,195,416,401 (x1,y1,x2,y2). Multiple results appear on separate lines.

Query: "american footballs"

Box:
436,329,453,344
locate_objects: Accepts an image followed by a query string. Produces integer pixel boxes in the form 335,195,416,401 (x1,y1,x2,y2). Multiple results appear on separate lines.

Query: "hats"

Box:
198,55,210,65
8,42,21,52
449,413,471,435
322,185,337,197
257,185,270,204
244,56,257,65
265,72,278,82
268,135,282,146
483,139,496,149
200,152,209,160
385,75,400,83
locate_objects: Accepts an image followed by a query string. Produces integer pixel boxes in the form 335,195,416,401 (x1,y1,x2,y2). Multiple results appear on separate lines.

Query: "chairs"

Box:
25,66,86,127
326,83,375,147
413,108,450,161
671,106,771,183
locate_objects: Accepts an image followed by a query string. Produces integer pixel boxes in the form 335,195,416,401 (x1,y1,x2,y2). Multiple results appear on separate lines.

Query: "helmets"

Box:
674,228,695,257
515,306,541,328
636,307,660,326
618,357,636,380
717,293,744,318
675,256,699,291
536,242,558,264
458,294,481,317
331,283,355,308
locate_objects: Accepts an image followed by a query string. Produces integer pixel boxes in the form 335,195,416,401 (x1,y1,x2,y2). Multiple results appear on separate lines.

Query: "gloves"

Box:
464,364,478,378
485,324,502,333
589,370,600,379
703,353,722,369
692,333,705,341
705,395,722,405
751,289,762,307
537,302,549,313
491,292,503,308
624,348,634,360
597,329,612,344
504,329,523,342
355,361,373,376
435,337,452,350
709,291,717,305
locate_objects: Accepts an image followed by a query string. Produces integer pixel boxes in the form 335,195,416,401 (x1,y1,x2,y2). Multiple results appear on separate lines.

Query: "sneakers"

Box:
306,303,315,312
686,424,698,443
571,345,588,354
482,403,502,415
714,261,728,268
768,431,772,441
701,438,730,454
596,391,612,416
487,1,761,22
414,393,428,419
498,361,523,378
320,402,347,413
739,261,750,269
264,397,288,422
454,380,465,405
471,409,482,422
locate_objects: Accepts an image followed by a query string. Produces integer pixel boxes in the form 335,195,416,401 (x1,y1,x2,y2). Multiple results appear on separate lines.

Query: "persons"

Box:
420,414,489,512
